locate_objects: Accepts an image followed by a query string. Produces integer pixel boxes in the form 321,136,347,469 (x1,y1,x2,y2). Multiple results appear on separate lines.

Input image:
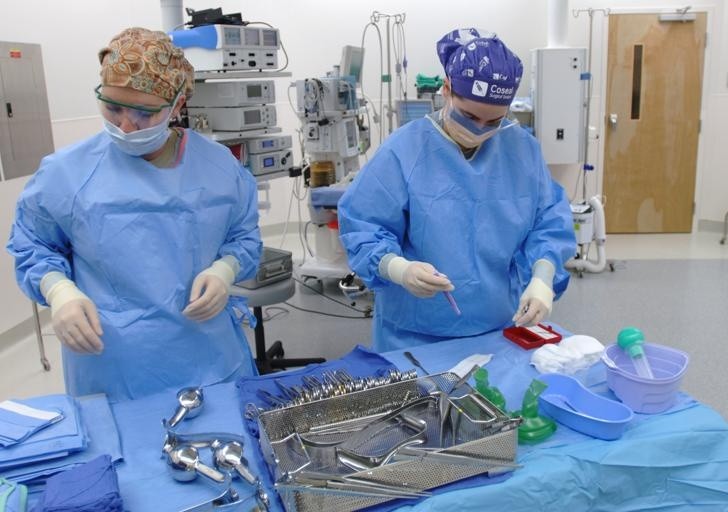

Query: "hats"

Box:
99,27,195,102
437,28,522,105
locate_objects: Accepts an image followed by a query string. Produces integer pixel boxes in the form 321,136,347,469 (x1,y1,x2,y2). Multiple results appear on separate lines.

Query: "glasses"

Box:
95,85,174,126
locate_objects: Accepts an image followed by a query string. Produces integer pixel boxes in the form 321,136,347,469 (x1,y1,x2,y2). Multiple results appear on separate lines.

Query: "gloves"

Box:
379,253,454,298
40,272,104,353
182,255,239,322
512,259,555,327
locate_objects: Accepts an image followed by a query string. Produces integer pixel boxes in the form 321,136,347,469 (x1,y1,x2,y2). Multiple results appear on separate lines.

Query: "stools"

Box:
228,279,326,372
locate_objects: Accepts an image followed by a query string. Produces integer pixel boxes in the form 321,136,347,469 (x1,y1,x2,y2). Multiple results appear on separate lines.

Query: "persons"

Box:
6,27,263,401
334,27,576,352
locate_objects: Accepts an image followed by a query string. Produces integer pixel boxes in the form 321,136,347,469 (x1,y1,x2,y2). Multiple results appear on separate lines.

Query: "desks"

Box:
0,320,727,512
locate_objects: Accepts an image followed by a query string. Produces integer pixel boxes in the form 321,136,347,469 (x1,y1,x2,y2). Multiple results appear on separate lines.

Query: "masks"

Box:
102,107,174,156
445,91,501,149
